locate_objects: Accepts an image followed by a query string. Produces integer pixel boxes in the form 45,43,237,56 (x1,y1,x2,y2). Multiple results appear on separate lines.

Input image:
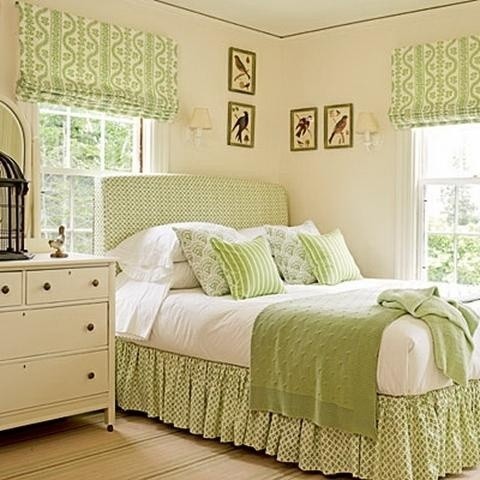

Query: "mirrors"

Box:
0,95,32,251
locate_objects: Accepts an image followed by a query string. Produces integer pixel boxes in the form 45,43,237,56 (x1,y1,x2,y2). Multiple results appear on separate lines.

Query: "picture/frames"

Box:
323,103,353,150
290,107,318,151
228,100,255,148
227,46,256,95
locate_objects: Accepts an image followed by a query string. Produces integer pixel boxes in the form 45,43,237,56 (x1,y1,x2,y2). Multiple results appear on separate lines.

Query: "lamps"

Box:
356,111,382,151
187,108,211,146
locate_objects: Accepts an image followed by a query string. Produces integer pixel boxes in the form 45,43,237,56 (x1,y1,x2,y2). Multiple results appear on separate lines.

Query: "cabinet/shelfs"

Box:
0,253,115,433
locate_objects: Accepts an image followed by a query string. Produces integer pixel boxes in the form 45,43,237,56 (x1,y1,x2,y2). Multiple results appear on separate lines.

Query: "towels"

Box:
378,288,480,389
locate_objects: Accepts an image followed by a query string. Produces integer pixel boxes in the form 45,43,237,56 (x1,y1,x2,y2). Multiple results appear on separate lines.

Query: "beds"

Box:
96,173,480,480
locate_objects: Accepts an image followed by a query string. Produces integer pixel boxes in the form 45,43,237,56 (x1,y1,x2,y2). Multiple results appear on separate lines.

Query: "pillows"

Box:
105,219,364,341
